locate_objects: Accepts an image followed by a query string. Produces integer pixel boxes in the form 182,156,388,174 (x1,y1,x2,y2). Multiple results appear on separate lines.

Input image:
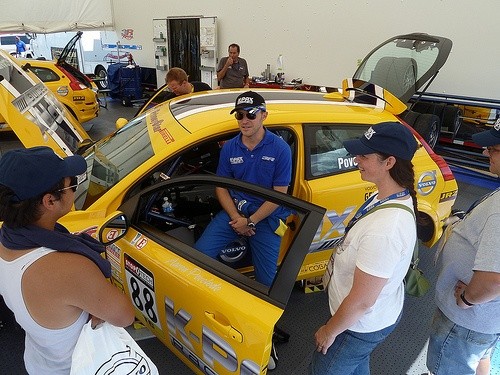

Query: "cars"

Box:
0,54,102,136
48,31,459,375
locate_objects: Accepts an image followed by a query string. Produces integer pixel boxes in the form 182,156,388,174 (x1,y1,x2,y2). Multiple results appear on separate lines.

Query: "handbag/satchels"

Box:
69,318,159,375
402,262,432,298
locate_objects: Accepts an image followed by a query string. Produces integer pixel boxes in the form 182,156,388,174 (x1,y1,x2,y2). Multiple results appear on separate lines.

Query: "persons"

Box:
16,37,28,54
164,67,212,100
216,44,250,88
308,122,418,375
426,113,500,375
195,91,292,369
0,147,134,375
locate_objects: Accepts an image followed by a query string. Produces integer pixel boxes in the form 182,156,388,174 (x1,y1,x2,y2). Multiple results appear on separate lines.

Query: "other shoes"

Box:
272,324,292,344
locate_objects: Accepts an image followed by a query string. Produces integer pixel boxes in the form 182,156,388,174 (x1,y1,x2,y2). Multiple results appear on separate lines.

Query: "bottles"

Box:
162,197,175,225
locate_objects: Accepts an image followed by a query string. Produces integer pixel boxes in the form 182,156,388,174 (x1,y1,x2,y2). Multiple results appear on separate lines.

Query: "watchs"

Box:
247,218,255,228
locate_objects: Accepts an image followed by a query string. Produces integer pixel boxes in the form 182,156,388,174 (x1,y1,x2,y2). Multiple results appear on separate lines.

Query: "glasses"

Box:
56,176,79,195
234,108,260,121
486,145,500,157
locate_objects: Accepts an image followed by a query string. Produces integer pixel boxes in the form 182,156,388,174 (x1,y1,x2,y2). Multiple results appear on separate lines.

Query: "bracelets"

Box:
460,292,473,306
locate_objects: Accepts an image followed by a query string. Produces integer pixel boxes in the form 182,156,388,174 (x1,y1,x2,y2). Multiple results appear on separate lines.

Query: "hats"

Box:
472,114,500,146
342,122,419,160
230,90,266,114
0,146,88,209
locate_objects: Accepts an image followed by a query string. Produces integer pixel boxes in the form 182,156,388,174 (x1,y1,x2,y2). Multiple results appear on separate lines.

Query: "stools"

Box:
99,89,111,108
